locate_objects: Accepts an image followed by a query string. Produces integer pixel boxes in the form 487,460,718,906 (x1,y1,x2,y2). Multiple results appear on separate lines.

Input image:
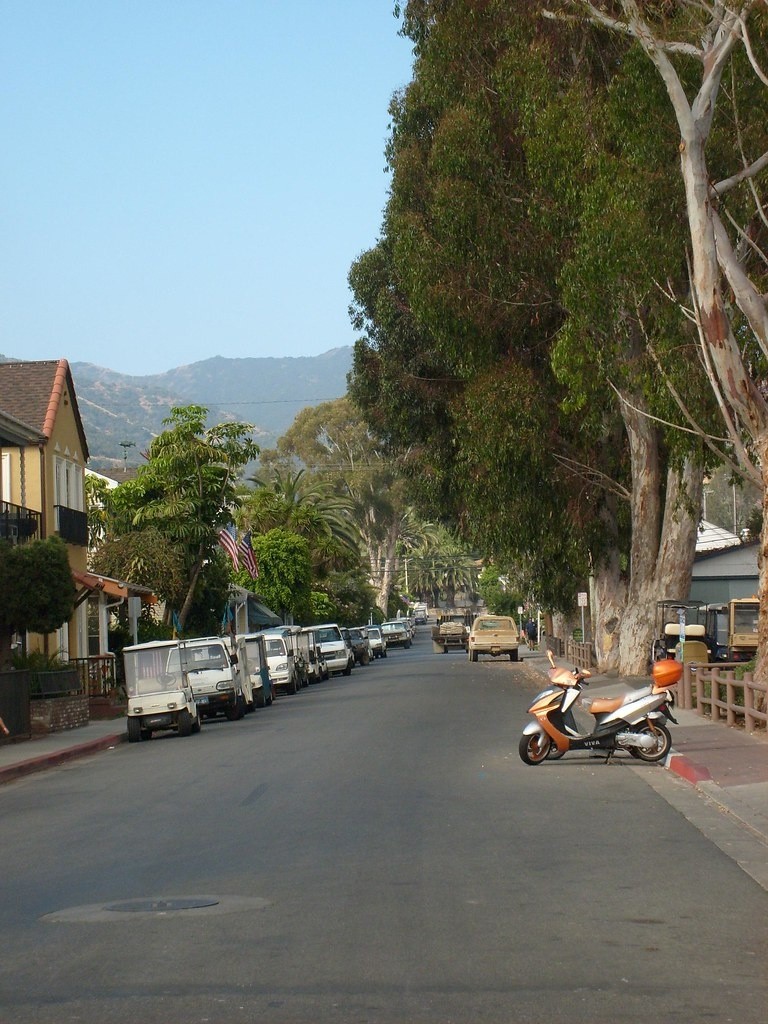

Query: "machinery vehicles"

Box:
649,594,760,673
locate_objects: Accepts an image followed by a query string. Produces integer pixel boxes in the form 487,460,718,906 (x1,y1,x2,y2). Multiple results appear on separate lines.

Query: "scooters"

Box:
519,650,684,766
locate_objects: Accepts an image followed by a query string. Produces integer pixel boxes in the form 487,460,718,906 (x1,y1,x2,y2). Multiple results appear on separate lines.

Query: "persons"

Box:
525,617,537,651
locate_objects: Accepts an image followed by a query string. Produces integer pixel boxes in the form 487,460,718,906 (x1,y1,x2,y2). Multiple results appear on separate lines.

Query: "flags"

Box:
403,594,410,605
222,606,233,625
237,531,259,580
216,519,239,573
173,612,183,633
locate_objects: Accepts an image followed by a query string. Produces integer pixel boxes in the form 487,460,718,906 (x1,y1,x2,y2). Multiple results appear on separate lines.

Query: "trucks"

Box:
298,604,429,683
233,634,274,707
164,636,253,720
259,626,310,696
430,614,475,653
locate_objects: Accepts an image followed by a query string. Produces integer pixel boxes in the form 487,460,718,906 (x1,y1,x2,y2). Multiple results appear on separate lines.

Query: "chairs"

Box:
269,642,279,654
207,648,222,665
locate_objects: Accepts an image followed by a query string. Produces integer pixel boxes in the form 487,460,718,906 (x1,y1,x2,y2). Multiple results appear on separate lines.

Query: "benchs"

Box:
665,623,707,650
138,673,187,694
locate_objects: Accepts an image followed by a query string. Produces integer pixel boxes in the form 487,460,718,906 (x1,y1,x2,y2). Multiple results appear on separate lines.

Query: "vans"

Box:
466,614,521,662
